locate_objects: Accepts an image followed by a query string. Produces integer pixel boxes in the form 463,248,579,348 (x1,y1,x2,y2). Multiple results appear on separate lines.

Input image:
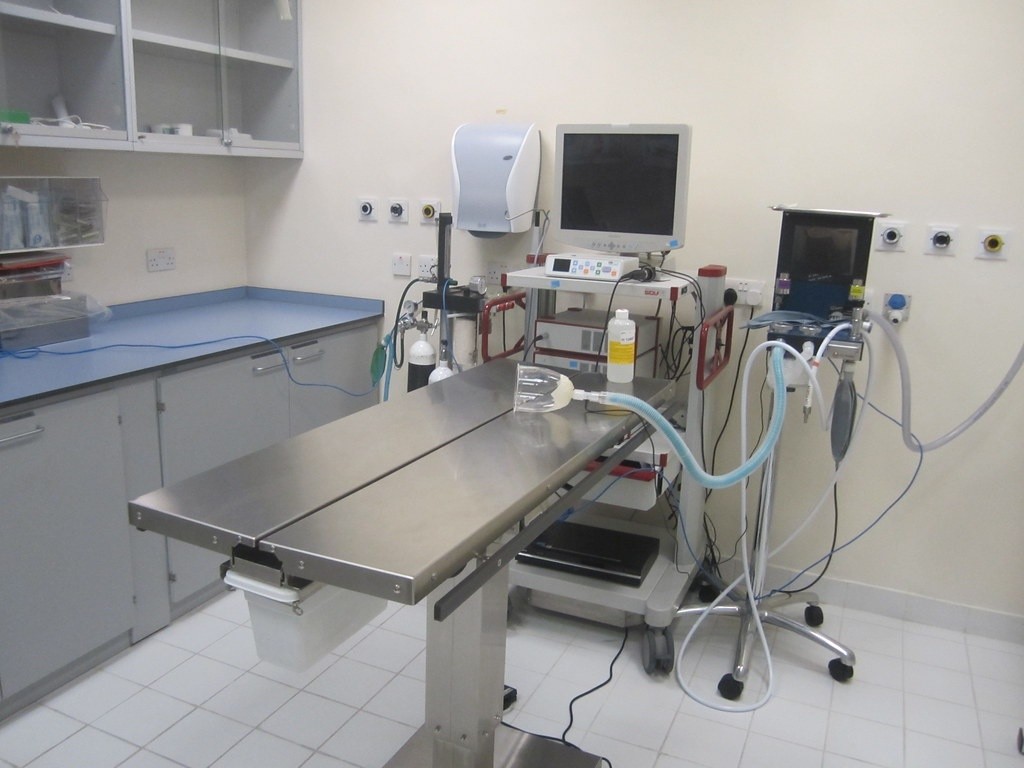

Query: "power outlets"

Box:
484,260,509,286
418,255,438,278
149,247,176,271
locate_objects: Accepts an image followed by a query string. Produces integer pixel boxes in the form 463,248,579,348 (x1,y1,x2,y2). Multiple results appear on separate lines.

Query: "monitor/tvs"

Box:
553,125,694,256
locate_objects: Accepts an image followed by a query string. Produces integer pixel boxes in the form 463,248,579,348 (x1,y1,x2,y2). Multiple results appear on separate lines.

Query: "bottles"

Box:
606,306,636,383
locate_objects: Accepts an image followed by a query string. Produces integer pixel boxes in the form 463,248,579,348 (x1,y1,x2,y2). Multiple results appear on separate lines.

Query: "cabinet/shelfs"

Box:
0,323,387,718
0,0,304,160
